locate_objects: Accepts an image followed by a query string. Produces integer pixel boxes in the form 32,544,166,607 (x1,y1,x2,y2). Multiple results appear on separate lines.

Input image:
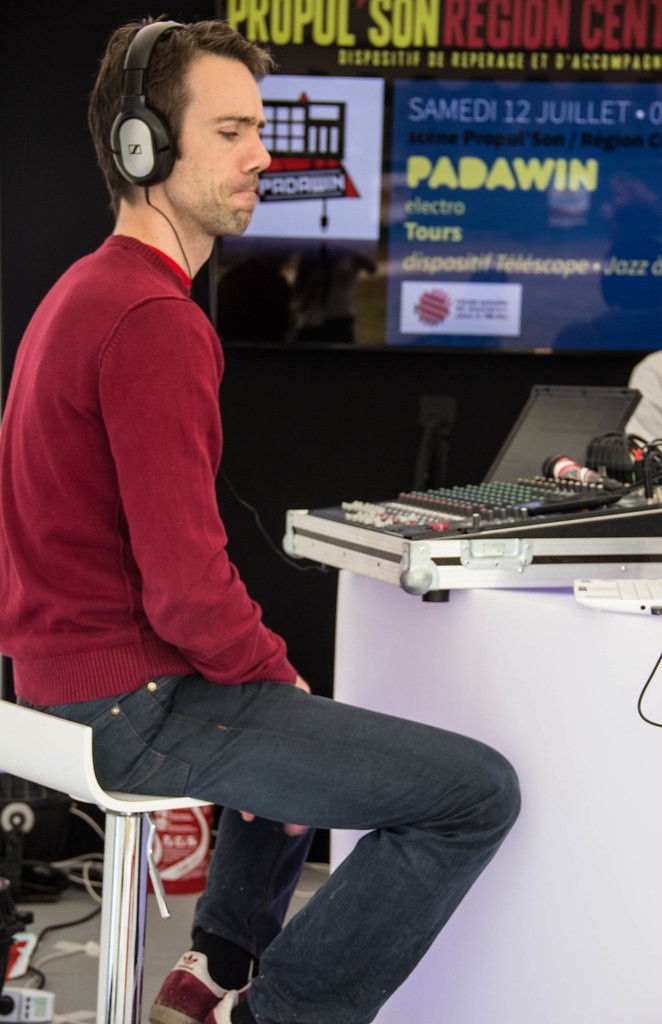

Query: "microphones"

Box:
542,455,626,490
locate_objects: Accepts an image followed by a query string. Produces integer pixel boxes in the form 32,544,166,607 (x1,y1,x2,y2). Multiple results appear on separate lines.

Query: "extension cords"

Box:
0,986,56,1022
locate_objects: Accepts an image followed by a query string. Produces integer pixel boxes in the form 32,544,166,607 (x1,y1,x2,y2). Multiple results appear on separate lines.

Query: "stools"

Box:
0,698,220,1024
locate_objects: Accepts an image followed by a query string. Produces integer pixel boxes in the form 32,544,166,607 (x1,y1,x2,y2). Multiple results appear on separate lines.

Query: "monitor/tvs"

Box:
210,0,662,353
478,384,644,485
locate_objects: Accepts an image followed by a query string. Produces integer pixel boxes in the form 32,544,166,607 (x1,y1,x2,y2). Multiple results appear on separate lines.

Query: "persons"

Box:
0,18,534,1022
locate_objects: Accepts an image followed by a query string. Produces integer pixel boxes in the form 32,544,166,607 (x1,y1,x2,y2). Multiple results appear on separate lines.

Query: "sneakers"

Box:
147,950,252,1024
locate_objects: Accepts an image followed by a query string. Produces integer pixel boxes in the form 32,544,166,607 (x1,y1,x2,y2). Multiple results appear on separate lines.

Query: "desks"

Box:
327,568,662,1024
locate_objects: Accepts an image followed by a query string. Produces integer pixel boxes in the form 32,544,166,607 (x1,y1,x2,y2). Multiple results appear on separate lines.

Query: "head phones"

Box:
109,20,189,186
